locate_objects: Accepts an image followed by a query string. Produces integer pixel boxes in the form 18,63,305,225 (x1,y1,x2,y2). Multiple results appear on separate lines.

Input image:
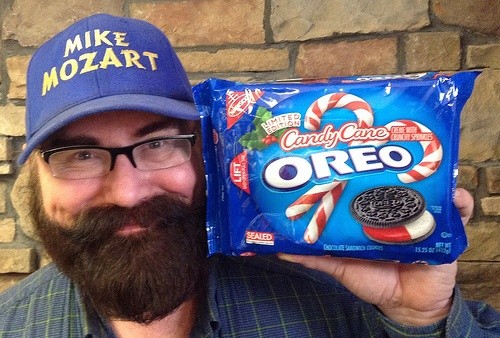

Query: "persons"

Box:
0,14,500,338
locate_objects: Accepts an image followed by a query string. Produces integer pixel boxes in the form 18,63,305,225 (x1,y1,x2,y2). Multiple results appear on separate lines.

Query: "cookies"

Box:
350,185,436,245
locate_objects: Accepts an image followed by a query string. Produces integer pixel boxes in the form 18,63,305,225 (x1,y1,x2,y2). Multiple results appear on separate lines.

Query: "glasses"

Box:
38,129,197,180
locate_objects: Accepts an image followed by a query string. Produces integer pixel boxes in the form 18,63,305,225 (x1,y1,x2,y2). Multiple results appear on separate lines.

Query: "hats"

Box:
17,13,201,164
